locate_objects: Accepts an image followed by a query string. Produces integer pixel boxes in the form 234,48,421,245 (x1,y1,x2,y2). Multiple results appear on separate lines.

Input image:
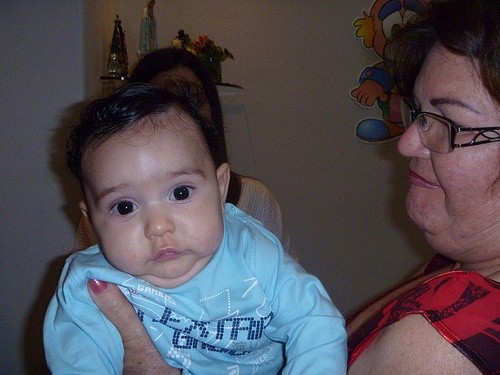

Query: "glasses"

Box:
399,96,500,154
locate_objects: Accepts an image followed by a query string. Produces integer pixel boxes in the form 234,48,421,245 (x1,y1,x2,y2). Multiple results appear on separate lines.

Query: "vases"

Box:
206,62,222,84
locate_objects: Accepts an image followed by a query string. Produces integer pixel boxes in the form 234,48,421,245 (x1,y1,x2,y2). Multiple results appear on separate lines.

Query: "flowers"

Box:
172,30,235,64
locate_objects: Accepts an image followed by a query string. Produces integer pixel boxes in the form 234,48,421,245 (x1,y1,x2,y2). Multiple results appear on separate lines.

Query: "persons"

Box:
116,47,301,269
83,0,499,375
39,76,349,375
